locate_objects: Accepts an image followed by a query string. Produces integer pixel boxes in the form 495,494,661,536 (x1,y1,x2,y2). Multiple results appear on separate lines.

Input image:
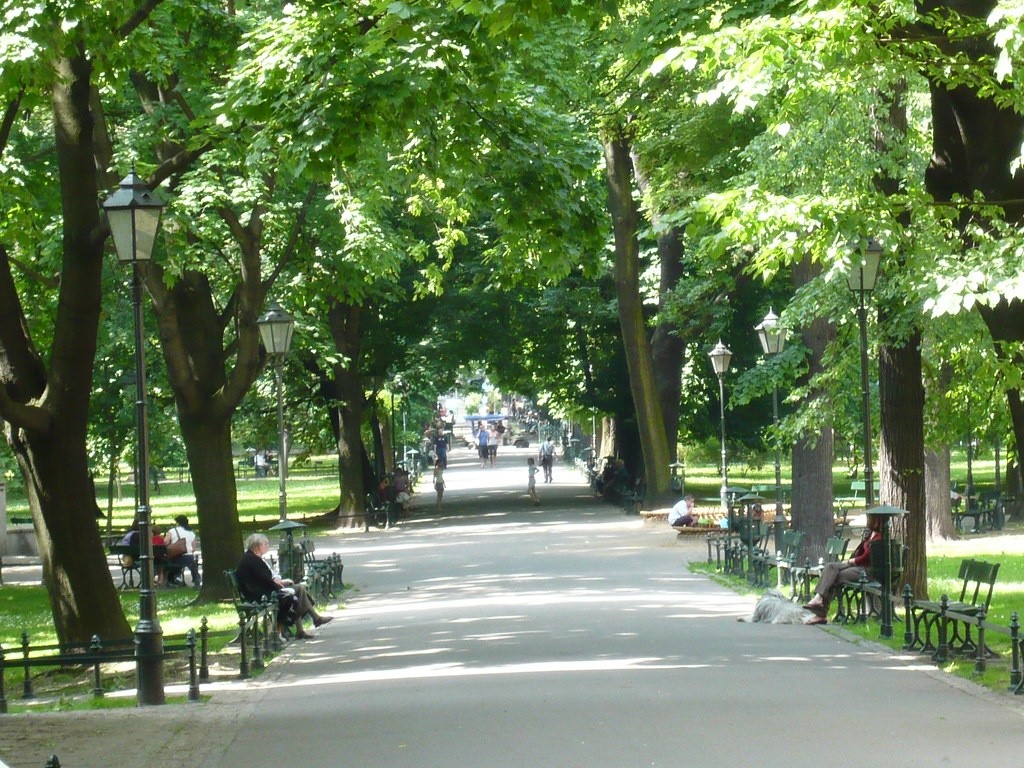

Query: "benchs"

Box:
365,459,423,528
952,491,1001,534
300,539,333,597
834,481,880,511
753,529,805,589
226,570,273,644
726,523,771,579
108,545,200,588
832,509,849,538
574,457,645,514
10,517,32,526
831,544,909,626
910,559,1001,661
950,481,963,530
790,537,851,605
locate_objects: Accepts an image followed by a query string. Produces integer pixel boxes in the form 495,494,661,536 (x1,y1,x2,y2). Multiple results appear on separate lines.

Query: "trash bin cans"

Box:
270,519,309,583
866,504,912,586
726,486,768,545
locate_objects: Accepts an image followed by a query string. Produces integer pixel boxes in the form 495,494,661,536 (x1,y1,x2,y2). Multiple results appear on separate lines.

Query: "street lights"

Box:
100,158,169,703
753,305,789,558
254,298,297,573
706,337,733,511
840,227,886,510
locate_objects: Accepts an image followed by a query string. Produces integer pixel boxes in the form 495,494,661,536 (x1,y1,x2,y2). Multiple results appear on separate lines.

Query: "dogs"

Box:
737,589,815,625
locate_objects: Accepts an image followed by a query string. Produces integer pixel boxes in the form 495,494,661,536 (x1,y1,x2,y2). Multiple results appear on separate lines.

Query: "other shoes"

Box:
802,602,824,610
295,630,314,641
193,573,201,585
314,616,333,628
168,578,180,585
805,615,827,626
281,627,294,637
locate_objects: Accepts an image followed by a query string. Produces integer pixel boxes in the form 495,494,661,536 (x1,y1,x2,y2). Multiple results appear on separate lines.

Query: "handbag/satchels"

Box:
165,527,187,560
849,532,872,559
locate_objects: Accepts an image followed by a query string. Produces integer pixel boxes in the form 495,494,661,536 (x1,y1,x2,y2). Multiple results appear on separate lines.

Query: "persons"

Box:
803,513,884,625
434,410,455,469
538,435,555,482
237,533,334,640
527,458,540,505
250,448,271,478
369,468,410,524
597,457,627,496
475,420,506,468
511,399,517,416
122,515,200,586
433,459,446,508
668,495,698,526
